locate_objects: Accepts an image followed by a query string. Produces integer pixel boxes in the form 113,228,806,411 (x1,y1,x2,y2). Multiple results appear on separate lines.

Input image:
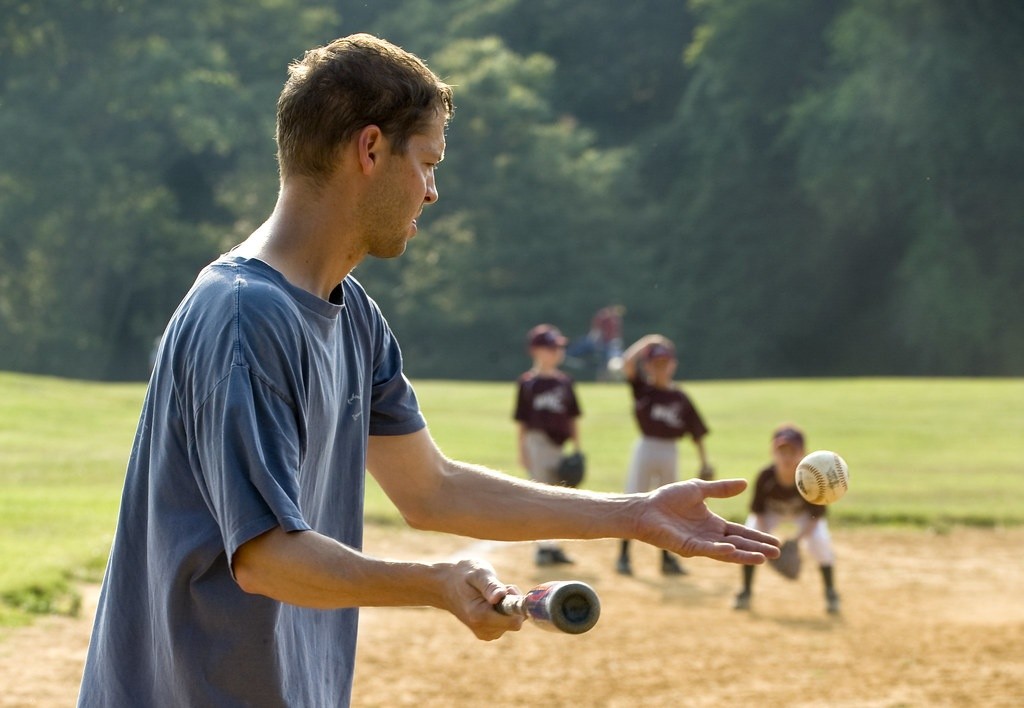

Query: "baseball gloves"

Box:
771,541,802,580
561,451,587,486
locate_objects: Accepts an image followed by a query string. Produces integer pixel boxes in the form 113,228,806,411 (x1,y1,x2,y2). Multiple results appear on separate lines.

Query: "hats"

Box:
646,345,679,360
526,323,571,348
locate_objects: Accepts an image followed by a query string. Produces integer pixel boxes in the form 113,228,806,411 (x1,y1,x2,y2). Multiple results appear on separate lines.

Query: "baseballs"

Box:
795,449,851,505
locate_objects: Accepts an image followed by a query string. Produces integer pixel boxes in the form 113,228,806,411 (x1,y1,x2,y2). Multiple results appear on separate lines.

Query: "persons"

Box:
732,424,843,618
613,331,710,580
512,324,588,574
590,304,623,384
75,31,782,707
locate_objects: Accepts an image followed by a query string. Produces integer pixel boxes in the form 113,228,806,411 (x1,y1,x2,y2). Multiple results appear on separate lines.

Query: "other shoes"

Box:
663,559,685,574
735,594,752,609
827,592,842,614
536,551,571,566
619,557,630,573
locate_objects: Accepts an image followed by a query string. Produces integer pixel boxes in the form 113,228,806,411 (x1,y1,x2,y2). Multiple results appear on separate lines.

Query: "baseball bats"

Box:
493,581,602,636
